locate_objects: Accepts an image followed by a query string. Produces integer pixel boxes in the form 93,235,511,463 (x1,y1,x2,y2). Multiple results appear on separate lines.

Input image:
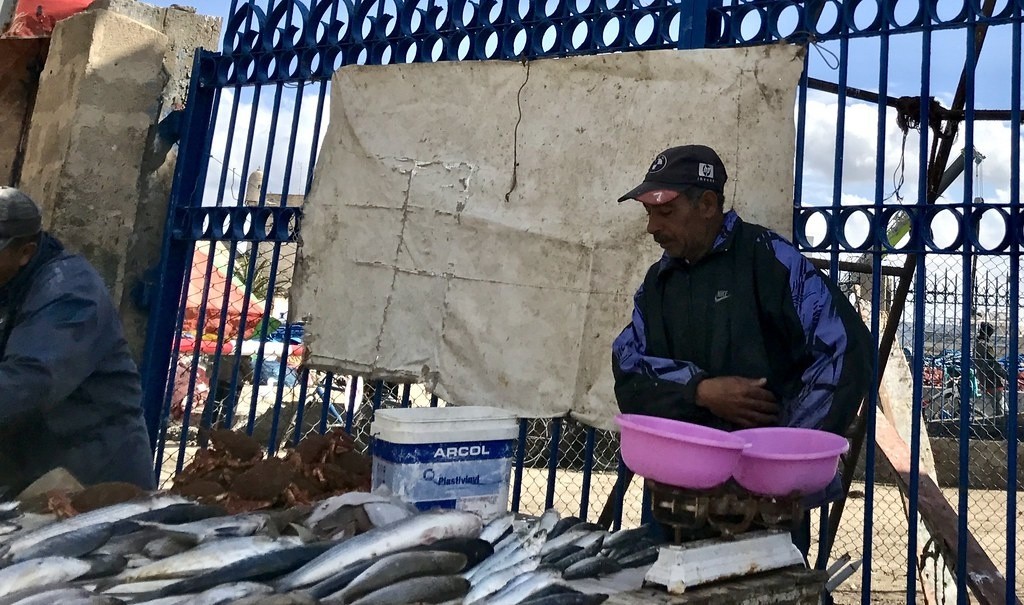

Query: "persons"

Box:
973,322,1009,416
196,355,254,446
0,186,158,504
612,146,872,558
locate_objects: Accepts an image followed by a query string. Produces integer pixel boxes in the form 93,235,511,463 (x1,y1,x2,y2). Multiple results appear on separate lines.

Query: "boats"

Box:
923,375,1024,444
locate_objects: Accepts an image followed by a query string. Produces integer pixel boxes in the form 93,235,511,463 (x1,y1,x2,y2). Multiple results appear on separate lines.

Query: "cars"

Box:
229,319,305,362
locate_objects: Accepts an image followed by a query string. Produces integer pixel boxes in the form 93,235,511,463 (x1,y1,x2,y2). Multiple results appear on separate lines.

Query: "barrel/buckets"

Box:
369,405,520,523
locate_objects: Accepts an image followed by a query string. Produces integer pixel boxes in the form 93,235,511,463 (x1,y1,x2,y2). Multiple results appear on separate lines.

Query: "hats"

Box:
0,185,43,251
617,145,728,206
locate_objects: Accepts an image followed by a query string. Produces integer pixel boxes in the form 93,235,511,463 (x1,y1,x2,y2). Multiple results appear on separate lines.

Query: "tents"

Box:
182,241,263,342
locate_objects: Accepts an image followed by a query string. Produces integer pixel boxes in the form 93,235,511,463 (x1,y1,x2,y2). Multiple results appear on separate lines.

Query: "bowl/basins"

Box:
728,426,849,496
613,413,752,489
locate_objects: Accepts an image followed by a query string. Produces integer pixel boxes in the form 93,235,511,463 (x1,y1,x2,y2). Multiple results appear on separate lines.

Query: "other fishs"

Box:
0,494,679,605
